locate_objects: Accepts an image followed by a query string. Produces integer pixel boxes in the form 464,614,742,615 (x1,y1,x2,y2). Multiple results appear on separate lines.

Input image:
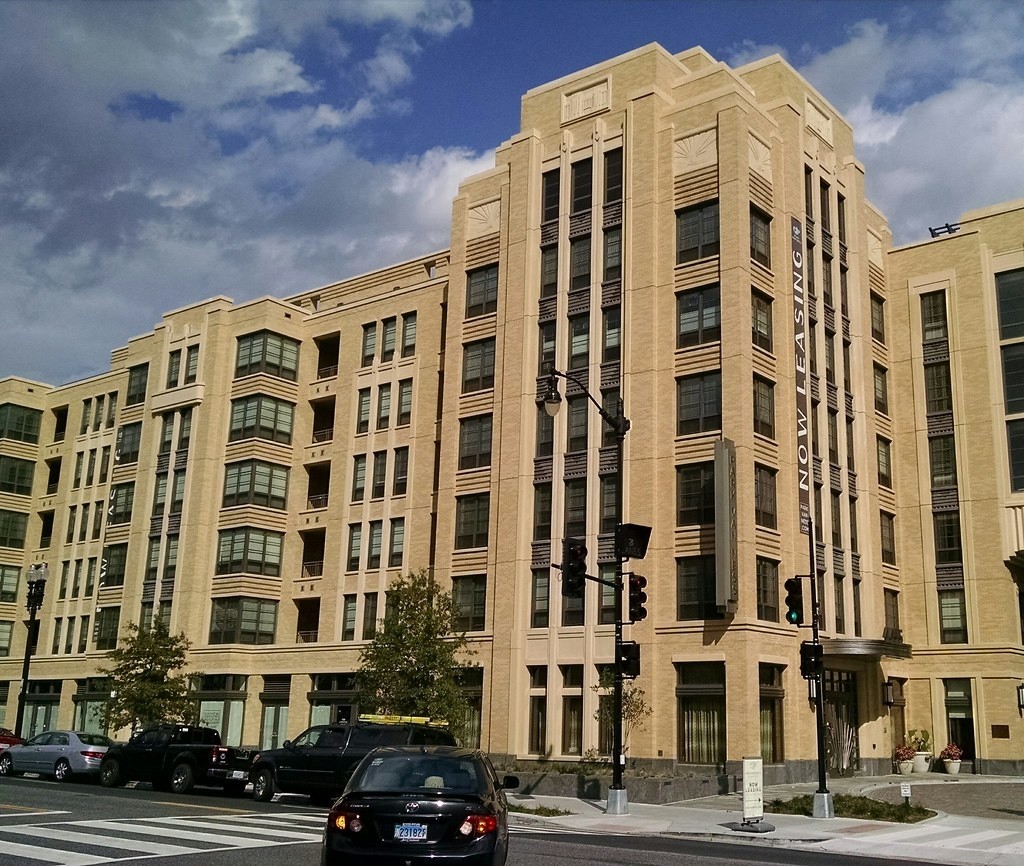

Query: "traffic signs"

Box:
741,757,764,820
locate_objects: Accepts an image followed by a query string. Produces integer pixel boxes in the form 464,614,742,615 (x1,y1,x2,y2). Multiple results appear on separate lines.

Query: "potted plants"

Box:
941,743,963,774
895,745,916,776
905,728,935,773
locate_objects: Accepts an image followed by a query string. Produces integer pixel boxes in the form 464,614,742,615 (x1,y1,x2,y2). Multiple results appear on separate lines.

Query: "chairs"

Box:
376,772,401,788
447,769,471,790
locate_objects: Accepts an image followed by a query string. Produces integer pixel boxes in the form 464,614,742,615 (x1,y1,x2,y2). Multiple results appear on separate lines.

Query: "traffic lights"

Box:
785,578,804,624
563,538,589,598
629,573,647,622
1003,550,1024,586
623,645,640,675
617,523,652,558
800,644,824,676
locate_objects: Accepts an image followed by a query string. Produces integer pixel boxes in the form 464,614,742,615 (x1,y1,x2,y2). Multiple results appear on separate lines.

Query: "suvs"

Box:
247,720,459,802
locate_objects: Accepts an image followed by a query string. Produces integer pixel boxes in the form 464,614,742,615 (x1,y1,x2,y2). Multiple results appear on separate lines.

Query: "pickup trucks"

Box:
99,724,261,796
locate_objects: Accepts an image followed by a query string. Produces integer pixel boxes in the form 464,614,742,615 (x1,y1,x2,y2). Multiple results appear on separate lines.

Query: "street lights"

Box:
14,562,49,737
542,367,632,814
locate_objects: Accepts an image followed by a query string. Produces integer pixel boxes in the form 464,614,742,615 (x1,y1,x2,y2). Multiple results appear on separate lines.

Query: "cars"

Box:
320,744,519,866
0,728,115,784
